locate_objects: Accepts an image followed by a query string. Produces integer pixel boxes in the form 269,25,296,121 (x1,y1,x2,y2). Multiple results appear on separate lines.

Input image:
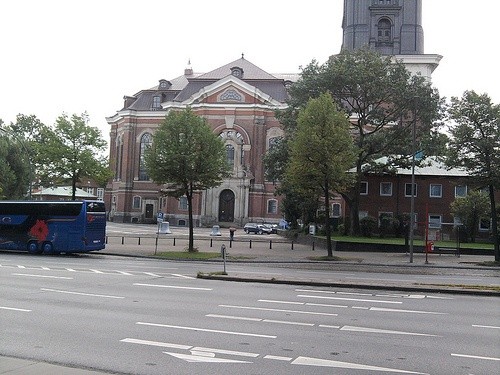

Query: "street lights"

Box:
409,148,423,265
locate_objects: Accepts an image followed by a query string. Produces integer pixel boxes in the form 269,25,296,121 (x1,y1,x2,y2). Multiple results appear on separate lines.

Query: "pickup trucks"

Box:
244,222,272,234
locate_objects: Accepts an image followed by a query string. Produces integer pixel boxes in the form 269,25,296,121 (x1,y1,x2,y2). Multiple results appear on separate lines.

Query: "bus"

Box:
0,200,106,255
